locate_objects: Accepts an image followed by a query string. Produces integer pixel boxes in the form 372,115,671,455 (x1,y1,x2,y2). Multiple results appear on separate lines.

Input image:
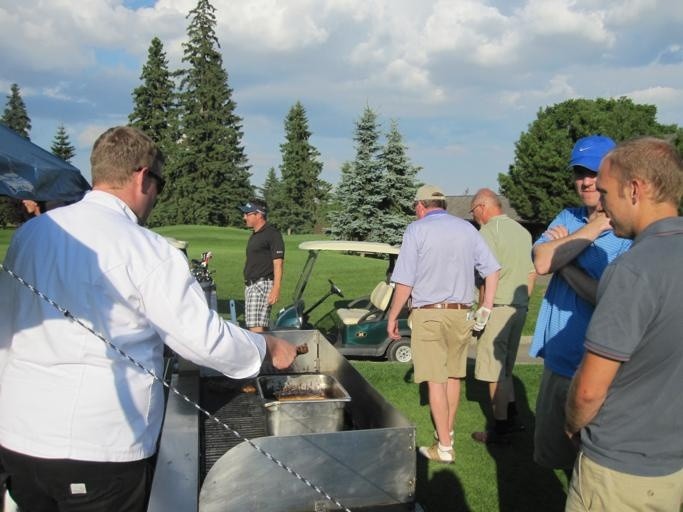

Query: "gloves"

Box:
473,306,491,331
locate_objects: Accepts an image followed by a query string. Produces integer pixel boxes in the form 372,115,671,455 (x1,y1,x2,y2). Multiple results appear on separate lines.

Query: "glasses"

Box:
468,204,481,215
133,167,166,194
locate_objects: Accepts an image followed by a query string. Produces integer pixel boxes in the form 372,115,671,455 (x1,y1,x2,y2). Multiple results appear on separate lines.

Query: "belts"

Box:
493,304,528,307
411,303,472,309
245,277,275,286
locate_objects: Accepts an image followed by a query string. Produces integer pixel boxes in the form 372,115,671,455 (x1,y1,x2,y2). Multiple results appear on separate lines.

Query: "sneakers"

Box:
472,431,510,448
434,430,454,446
506,415,526,433
419,444,455,464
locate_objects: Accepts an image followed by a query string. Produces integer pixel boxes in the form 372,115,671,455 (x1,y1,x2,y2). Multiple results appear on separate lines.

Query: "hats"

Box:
238,201,266,214
568,136,616,173
414,184,446,201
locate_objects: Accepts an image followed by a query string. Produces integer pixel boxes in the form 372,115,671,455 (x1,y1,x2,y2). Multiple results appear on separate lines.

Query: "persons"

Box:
22,198,46,217
563,137,683,511
0,125,297,512
528,135,634,470
386,184,502,463
470,188,536,444
236,198,285,330
